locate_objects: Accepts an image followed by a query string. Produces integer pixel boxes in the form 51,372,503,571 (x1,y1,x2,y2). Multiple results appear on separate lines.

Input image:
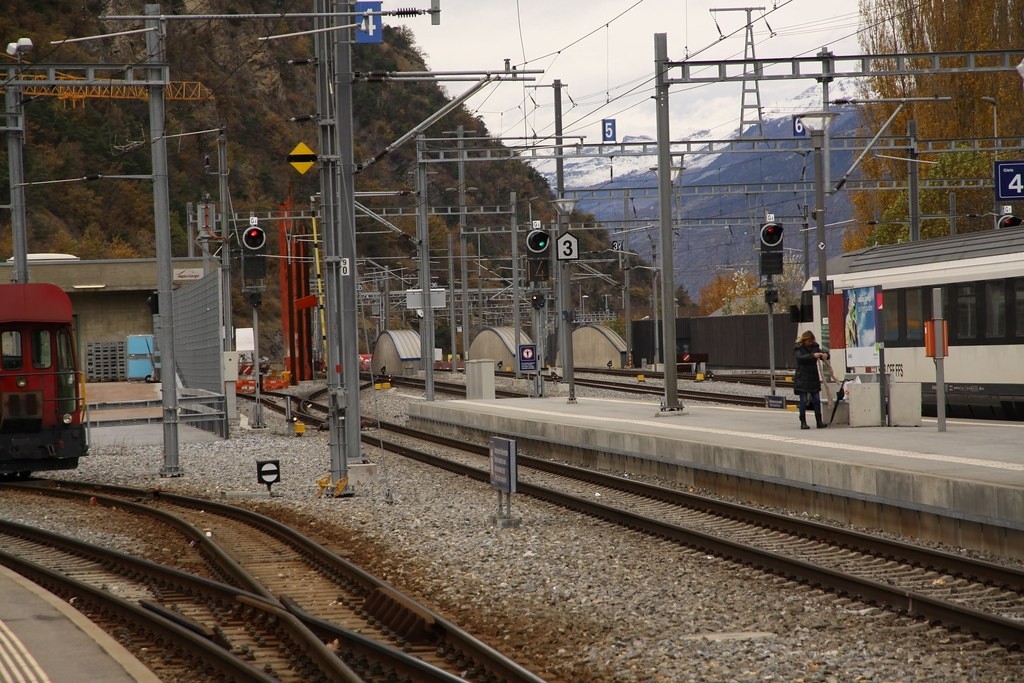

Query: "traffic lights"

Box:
241,226,268,282
524,226,555,285
761,223,784,277
998,214,1022,229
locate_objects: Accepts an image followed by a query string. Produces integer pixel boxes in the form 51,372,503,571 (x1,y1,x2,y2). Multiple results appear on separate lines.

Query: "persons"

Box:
794,331,830,429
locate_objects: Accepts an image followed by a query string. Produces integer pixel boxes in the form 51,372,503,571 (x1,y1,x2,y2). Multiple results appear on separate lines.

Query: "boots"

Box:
799,415,810,429
815,414,827,428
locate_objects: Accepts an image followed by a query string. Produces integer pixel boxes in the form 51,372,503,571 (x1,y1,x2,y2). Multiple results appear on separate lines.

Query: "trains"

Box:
792,224,1024,417
0,282,91,481
